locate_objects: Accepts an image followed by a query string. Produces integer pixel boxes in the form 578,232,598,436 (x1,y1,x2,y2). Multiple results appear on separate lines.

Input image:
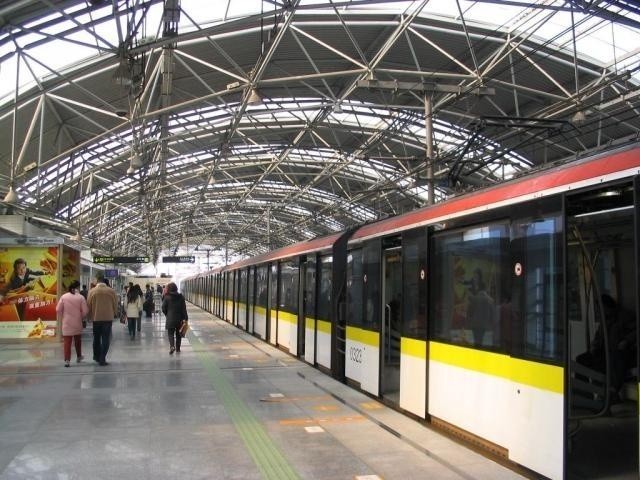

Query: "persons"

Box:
0,257,50,321
162,283,188,354
121,281,169,341
568,296,639,421
56,280,90,368
86,278,118,366
80,285,88,327
452,265,525,354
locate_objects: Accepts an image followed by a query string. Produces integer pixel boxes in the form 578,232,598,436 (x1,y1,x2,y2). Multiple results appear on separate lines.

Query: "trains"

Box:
179,133,640,480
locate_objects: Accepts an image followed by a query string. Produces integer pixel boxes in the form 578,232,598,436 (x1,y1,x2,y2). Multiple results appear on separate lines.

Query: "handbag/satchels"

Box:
179,320,188,338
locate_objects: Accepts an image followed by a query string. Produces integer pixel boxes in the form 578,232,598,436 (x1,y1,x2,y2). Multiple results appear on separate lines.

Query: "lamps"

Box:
209,174,216,185
246,88,261,107
131,152,143,168
0,186,21,205
111,60,133,85
